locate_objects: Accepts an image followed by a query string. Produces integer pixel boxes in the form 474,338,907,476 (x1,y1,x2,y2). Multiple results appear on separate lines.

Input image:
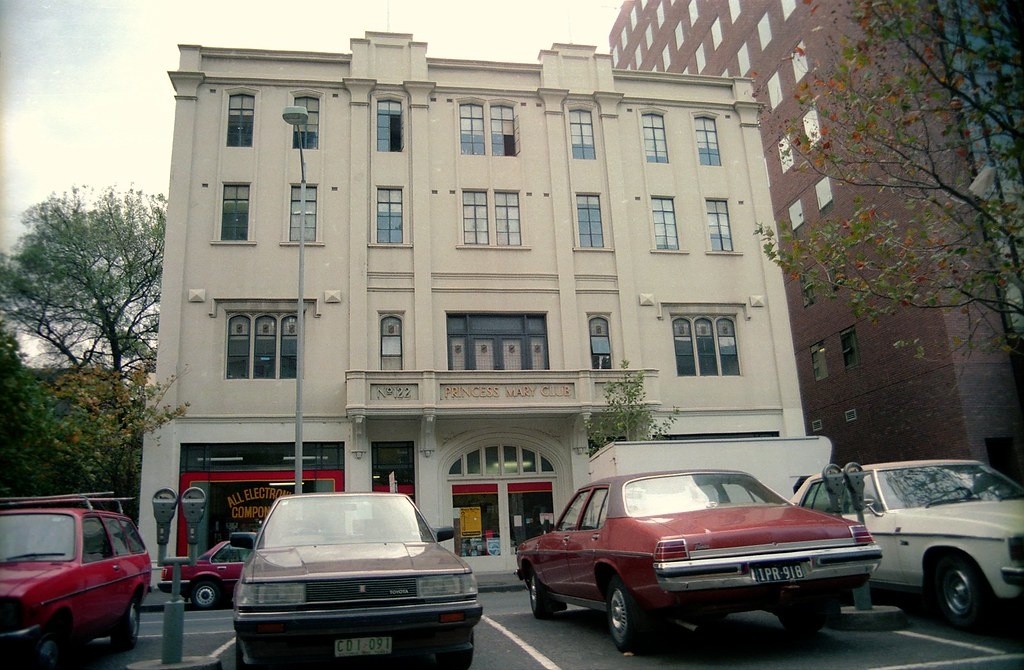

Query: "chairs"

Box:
278,500,417,543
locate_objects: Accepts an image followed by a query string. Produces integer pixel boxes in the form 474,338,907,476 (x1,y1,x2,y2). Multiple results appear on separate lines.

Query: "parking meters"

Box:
151,487,207,664
820,462,874,612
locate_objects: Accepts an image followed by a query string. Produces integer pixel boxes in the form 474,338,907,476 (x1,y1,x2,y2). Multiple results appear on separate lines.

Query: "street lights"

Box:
281,106,310,494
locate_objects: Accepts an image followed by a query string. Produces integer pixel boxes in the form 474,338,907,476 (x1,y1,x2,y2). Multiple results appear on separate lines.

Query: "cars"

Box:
157,539,253,610
784,459,1024,632
0,508,153,670
513,467,883,656
230,491,484,670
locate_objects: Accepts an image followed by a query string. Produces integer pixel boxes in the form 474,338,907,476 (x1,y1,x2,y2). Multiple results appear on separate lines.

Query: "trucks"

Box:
587,435,834,525
